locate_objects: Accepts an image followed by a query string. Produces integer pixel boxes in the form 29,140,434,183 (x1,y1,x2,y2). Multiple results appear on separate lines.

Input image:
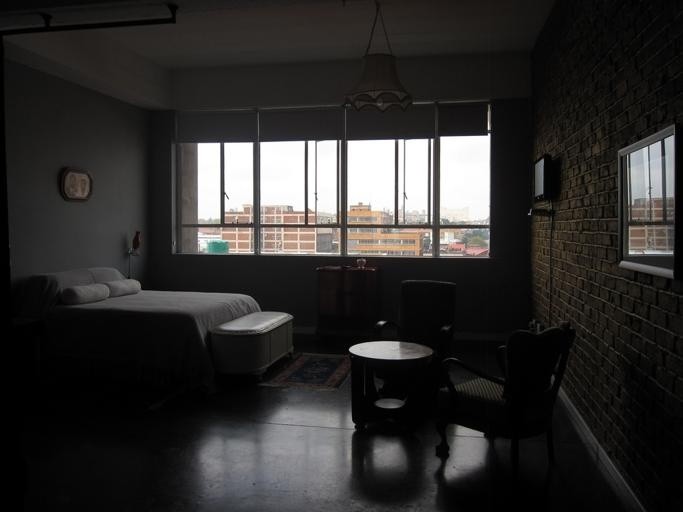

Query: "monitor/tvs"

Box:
533,153,552,202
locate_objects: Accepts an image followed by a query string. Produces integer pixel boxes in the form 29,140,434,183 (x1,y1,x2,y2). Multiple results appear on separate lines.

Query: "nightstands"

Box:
12,314,44,398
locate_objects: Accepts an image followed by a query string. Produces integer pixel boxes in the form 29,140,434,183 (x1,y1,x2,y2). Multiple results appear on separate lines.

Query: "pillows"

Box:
26,267,124,303
62,283,110,304
103,278,141,298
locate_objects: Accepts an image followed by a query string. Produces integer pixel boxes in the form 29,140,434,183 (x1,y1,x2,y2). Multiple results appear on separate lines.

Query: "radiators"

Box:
316,264,382,335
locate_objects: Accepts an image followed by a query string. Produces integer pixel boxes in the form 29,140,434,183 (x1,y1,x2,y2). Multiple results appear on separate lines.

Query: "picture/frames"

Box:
61,168,93,201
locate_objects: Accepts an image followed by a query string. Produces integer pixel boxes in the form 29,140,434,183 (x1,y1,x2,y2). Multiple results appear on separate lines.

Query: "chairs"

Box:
435,327,576,479
376,279,456,403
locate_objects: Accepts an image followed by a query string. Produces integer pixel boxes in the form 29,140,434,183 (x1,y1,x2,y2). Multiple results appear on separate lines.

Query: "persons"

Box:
66,173,77,197
75,178,88,197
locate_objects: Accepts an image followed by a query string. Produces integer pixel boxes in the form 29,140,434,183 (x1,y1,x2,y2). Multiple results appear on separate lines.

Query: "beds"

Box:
22,267,262,399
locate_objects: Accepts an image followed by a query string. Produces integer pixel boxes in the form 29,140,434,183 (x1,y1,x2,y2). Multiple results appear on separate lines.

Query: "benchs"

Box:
210,310,295,380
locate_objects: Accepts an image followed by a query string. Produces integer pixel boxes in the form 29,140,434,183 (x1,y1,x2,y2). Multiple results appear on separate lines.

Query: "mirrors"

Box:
617,122,677,280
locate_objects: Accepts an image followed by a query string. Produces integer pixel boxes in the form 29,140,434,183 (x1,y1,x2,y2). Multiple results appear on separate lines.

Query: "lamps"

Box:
339,0,411,113
127,231,141,279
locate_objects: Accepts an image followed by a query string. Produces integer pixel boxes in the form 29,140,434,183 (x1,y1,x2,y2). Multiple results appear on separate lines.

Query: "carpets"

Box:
259,351,350,392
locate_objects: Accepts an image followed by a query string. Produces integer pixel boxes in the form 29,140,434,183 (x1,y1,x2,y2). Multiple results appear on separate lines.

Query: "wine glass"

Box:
357,258,367,270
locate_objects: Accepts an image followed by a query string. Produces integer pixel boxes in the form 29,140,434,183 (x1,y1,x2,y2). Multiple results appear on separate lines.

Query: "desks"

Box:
348,341,434,437
